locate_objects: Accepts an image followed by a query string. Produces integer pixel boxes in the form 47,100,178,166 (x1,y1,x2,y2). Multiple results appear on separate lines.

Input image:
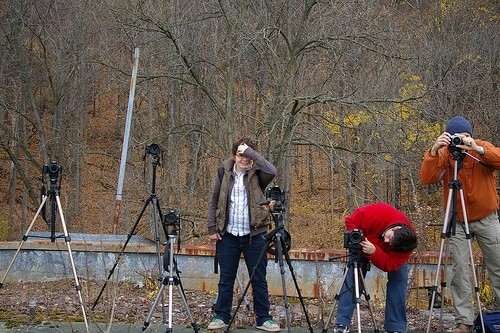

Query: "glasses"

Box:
236,154,251,159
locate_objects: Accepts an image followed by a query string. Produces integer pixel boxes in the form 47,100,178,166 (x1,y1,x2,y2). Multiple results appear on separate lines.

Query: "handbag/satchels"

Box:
474,311,500,333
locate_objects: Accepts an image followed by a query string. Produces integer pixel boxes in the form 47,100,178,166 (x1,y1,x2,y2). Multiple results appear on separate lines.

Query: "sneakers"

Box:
256,320,280,331
208,318,229,330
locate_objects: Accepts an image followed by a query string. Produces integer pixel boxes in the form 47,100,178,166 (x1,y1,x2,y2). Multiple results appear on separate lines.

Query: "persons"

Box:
334,204,419,333
207,138,280,331
420,116,500,333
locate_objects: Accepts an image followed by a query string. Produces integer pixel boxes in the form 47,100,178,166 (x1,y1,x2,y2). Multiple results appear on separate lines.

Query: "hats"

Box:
445,116,473,138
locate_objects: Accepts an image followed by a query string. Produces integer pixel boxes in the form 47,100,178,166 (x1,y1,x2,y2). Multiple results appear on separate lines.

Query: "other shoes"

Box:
446,324,472,333
333,324,350,333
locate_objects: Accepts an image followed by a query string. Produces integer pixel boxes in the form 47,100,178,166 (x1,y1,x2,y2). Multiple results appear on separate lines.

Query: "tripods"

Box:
322,251,380,332
140,225,200,333
90,161,191,324
426,150,496,333
1,181,91,333
222,204,314,333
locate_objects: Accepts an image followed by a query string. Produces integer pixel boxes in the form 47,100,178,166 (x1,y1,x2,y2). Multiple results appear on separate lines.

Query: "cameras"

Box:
163,209,180,233
447,133,466,148
267,187,284,207
42,160,63,184
144,141,160,163
344,227,363,248
